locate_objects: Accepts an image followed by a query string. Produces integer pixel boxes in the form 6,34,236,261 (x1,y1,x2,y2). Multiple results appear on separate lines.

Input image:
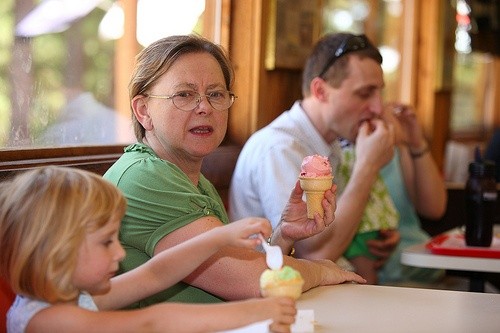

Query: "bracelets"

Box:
268,233,296,257
409,141,432,158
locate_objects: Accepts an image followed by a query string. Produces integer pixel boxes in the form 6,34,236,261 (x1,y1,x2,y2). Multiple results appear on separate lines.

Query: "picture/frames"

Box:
265,0,320,70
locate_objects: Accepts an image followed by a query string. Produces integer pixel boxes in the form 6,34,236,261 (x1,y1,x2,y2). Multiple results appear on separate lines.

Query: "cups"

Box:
465,161,495,247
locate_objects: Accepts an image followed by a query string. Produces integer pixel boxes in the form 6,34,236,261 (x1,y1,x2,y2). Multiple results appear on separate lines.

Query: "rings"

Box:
325,224,329,226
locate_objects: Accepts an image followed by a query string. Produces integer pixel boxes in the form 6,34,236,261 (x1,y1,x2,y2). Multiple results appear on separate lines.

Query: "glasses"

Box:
317,33,374,79
144,90,238,113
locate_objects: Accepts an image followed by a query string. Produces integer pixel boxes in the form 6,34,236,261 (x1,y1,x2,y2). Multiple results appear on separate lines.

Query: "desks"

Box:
401,225,500,272
218,283,500,333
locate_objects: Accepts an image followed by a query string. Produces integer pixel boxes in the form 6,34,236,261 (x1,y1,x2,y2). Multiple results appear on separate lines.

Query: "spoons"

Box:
258,234,283,269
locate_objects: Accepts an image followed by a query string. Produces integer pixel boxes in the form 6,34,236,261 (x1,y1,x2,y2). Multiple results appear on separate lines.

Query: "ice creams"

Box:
260,266,305,333
298,153,334,219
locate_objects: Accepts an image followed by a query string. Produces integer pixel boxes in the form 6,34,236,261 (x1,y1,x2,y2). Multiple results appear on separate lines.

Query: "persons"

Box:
228,31,401,285
100,34,368,309
337,100,449,292
0,164,297,333
